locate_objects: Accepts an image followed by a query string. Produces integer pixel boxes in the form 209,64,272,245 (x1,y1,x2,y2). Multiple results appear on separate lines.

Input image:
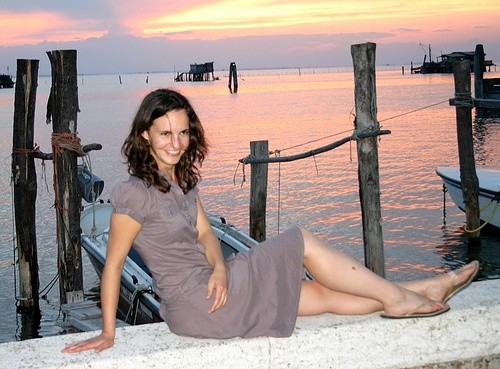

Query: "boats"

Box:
77,199,314,325
435,166,500,230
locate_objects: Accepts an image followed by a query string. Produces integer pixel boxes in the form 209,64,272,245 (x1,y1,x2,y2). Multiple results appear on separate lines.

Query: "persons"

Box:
60,88,479,353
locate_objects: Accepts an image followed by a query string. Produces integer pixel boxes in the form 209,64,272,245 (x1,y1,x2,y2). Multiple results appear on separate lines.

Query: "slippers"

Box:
380,300,450,319
443,260,480,303
